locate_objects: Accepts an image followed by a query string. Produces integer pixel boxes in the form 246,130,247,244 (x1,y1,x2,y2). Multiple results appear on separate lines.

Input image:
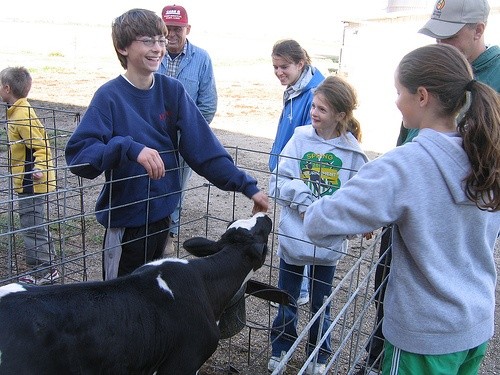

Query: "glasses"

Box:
131,37,168,47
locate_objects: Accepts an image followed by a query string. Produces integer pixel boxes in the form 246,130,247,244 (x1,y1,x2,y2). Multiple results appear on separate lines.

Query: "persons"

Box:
271,40,325,307
153,4,218,254
0,66,59,286
305,44,500,375
66,10,271,282
269,76,375,375
355,0,500,375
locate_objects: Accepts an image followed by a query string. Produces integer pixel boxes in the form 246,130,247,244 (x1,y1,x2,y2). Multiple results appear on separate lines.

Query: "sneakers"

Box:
19,268,60,285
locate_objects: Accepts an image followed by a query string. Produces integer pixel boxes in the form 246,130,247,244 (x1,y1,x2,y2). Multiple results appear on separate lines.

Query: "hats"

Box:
162,4,188,26
417,0,490,40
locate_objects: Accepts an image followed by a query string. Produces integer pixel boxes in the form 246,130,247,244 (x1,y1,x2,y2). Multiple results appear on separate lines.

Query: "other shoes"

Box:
305,361,326,375
267,351,294,372
269,292,310,308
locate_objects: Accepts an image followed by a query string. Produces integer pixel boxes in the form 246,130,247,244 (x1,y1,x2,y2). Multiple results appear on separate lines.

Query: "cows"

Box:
0,212,273,375
